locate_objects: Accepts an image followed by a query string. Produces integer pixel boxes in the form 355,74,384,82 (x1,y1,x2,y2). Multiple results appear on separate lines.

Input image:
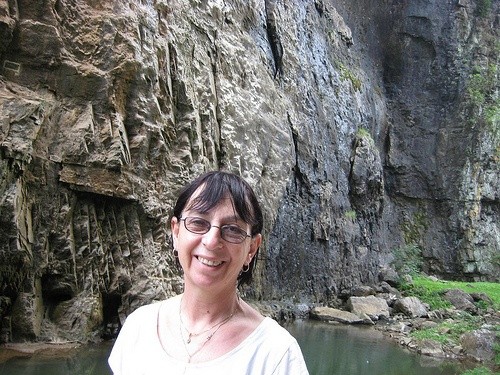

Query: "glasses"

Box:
177,215,256,245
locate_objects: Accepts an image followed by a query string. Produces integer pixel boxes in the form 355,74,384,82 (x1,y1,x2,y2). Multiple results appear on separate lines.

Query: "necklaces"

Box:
179,294,240,364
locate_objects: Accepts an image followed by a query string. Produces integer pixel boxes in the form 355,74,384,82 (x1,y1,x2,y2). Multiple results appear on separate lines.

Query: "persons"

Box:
107,171,309,375
